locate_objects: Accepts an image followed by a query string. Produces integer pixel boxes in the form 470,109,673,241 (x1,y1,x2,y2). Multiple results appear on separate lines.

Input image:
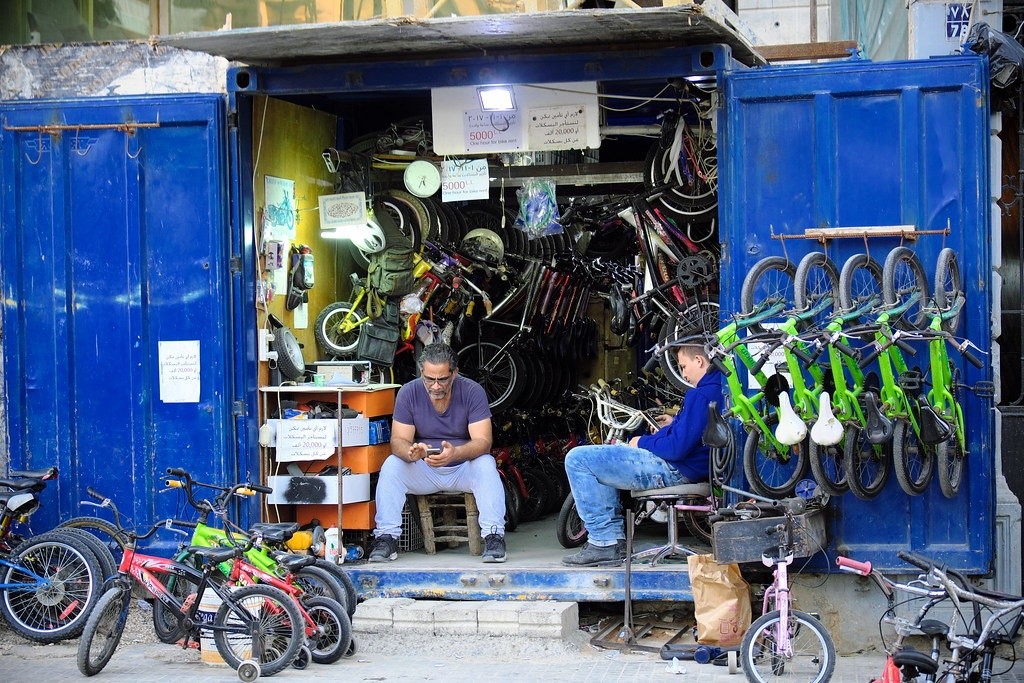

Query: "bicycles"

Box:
0,116,1024,682
739,522,838,683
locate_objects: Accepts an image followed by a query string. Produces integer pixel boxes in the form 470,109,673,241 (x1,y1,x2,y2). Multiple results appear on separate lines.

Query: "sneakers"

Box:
368,534,398,562
482,525,507,563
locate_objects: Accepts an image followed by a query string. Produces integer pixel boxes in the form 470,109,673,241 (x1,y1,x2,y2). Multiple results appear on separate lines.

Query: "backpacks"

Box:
367,201,413,319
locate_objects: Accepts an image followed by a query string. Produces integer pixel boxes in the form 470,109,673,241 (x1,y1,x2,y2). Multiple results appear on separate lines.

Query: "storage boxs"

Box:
711,510,828,566
316,364,365,384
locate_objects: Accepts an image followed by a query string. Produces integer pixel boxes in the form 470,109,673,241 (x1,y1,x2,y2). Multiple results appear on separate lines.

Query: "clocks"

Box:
403,158,442,199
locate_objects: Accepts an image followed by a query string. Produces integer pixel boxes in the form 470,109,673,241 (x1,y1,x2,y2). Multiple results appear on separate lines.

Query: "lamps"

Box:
475,84,516,113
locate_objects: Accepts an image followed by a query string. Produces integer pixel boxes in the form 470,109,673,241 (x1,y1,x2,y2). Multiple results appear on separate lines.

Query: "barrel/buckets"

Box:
198,586,262,664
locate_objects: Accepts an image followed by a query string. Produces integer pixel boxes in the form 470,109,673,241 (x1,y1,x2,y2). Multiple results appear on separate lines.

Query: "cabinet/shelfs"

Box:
262,383,402,563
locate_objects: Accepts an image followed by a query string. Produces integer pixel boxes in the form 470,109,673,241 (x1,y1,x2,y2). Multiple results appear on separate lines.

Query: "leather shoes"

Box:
561,539,632,568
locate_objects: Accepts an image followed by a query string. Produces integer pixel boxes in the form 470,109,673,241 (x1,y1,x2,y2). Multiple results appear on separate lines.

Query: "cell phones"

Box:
640,411,662,433
427,448,440,459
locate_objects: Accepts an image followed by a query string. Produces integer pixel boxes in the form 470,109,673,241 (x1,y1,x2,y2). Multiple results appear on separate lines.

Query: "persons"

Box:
367,342,508,563
561,338,728,568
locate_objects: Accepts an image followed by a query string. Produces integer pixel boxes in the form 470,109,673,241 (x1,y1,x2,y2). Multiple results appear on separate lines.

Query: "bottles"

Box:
324,523,344,564
302,249,314,284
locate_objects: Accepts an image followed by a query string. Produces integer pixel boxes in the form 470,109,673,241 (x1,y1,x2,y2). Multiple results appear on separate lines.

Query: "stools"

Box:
416,492,482,557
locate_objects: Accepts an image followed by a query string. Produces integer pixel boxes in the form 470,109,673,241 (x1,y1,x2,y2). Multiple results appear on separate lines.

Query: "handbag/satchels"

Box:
357,321,400,367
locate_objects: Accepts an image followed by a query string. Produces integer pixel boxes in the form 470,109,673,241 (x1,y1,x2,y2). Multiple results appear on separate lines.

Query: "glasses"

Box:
422,372,452,385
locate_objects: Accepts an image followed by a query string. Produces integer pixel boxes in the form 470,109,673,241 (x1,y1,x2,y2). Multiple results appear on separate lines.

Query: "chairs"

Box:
623,481,715,567
